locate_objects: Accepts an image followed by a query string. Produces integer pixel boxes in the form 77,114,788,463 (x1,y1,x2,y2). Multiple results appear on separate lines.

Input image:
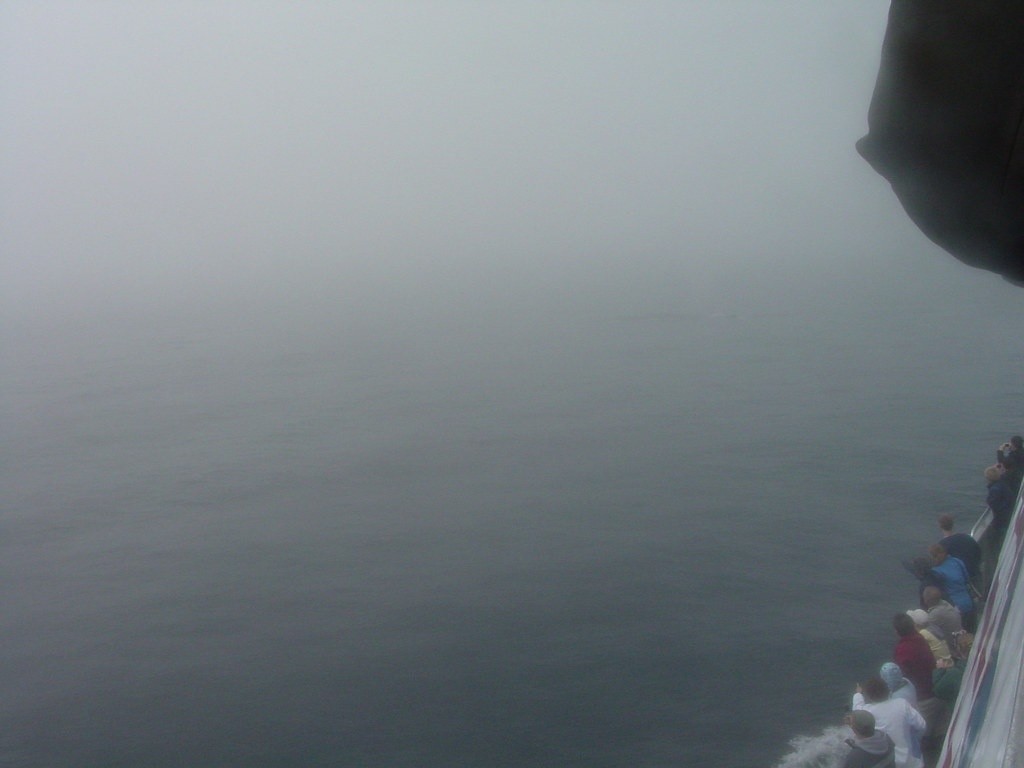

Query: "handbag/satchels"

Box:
966,580,982,603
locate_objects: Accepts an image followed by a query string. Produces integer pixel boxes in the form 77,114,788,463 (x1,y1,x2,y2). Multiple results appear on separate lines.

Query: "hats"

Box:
879,663,902,686
906,609,930,626
984,466,1000,482
851,710,876,735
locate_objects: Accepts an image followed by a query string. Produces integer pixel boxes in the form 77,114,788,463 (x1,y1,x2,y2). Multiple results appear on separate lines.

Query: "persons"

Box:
845,436,1024,766
935,516,982,596
